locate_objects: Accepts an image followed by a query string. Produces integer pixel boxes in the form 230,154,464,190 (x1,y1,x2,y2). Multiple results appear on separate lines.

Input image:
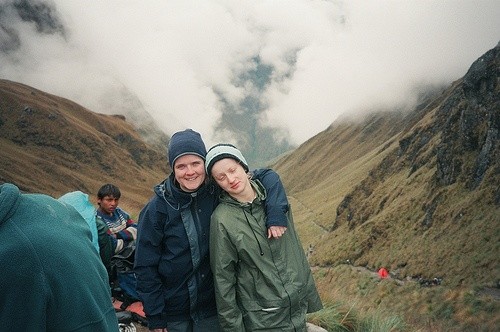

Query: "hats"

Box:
167,128,207,172
205,143,249,177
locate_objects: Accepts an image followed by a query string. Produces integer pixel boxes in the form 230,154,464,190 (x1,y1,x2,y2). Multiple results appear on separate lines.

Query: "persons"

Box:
0,183,121,332
94,184,140,302
133,129,287,332
204,144,325,332
307,243,313,254
